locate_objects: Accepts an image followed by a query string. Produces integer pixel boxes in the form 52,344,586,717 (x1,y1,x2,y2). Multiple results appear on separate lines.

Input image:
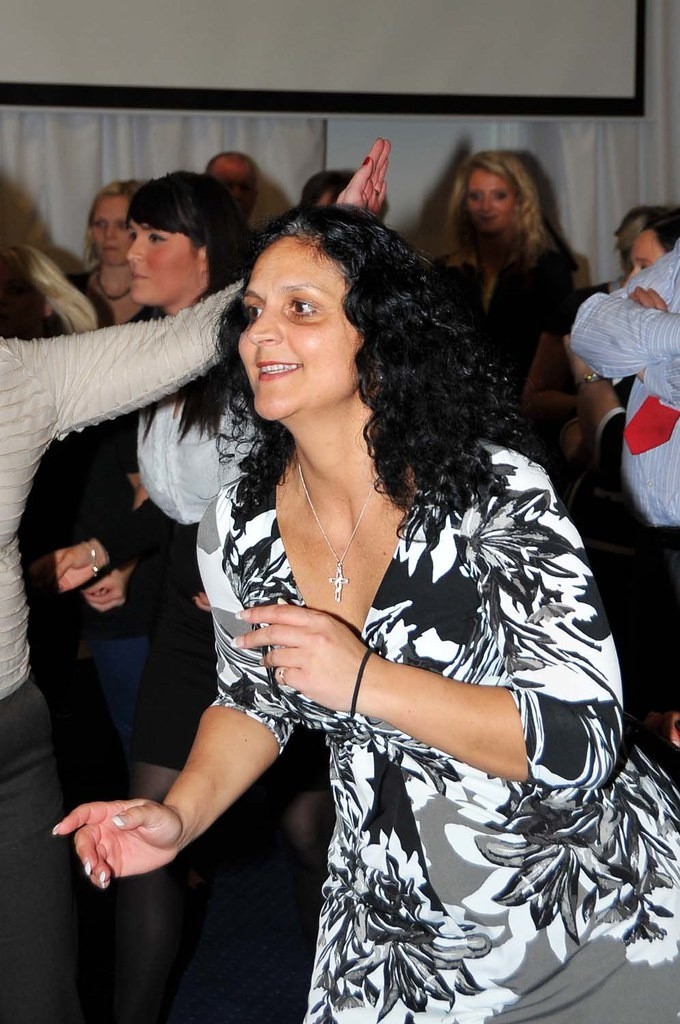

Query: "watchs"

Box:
575,374,609,387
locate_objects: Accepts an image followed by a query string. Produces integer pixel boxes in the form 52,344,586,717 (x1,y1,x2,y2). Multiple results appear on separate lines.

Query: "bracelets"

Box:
81,541,99,577
350,648,374,718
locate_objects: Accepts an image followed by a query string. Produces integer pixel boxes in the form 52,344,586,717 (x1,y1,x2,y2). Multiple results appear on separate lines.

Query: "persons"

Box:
0,138,391,1024
301,172,352,206
563,208,680,467
434,149,578,390
205,151,258,221
568,238,680,711
521,206,680,410
0,170,336,1024
53,206,680,1024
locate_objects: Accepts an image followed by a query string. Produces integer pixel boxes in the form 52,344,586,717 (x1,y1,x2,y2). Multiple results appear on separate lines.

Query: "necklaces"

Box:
298,463,378,602
95,269,130,300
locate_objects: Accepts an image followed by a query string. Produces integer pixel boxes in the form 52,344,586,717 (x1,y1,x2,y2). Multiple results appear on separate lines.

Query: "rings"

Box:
279,667,289,685
374,189,380,196
